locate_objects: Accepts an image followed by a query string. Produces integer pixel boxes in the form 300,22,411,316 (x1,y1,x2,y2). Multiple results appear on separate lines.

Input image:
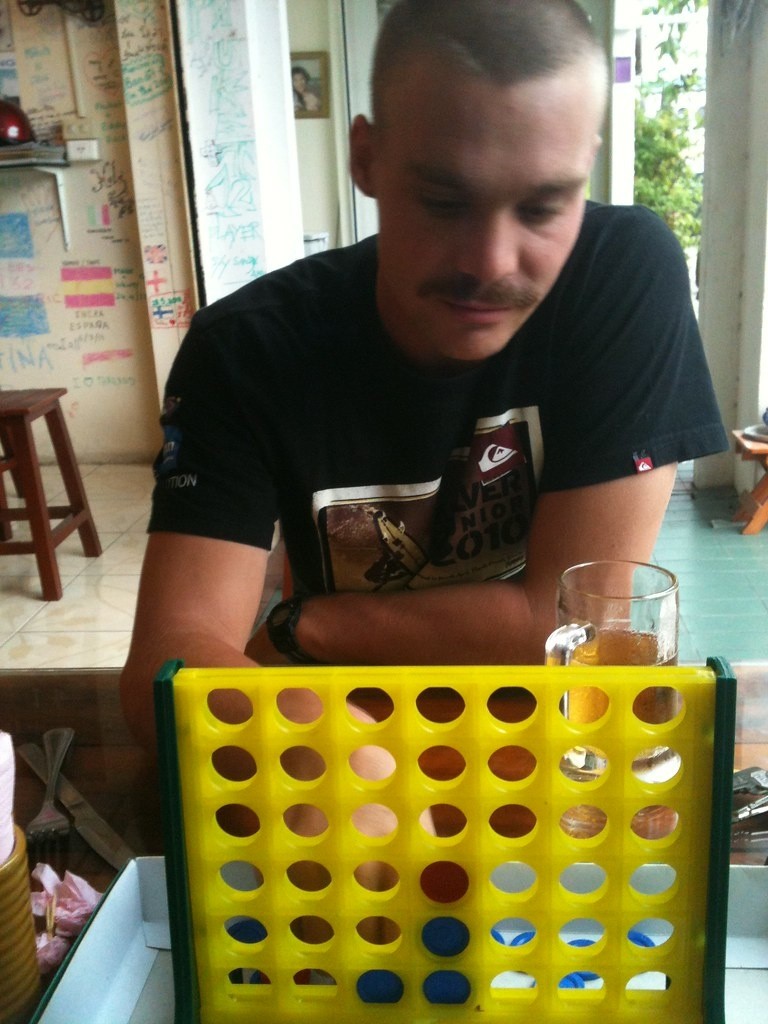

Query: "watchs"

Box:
267,593,325,666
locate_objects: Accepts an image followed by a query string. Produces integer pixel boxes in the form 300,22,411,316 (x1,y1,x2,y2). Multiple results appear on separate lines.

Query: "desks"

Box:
0,659,768,1024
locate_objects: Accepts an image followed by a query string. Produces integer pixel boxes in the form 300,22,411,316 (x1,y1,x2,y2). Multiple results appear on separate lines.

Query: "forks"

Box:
26,727,75,842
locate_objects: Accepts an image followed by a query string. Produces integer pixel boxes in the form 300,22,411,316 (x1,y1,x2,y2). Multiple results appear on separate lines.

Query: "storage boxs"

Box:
30,855,768,1024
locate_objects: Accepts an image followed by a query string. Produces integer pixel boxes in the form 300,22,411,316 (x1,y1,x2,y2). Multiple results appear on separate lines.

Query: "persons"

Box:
124,0,731,944
291,66,322,112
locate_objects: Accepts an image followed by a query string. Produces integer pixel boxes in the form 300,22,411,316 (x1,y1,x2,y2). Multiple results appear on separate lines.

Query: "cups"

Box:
0,822,40,1024
545,559,678,838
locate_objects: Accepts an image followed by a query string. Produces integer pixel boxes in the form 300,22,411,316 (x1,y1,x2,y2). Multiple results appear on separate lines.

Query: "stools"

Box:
731,430,768,535
0,388,104,601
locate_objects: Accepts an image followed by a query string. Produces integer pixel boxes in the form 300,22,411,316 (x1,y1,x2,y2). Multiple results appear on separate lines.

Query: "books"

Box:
0,142,65,166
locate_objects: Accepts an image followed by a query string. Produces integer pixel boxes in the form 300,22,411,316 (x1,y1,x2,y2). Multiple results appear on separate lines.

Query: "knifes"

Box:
17,741,137,871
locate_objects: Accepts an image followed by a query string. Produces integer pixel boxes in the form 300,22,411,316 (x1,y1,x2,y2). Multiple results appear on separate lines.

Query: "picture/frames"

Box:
291,50,331,120
